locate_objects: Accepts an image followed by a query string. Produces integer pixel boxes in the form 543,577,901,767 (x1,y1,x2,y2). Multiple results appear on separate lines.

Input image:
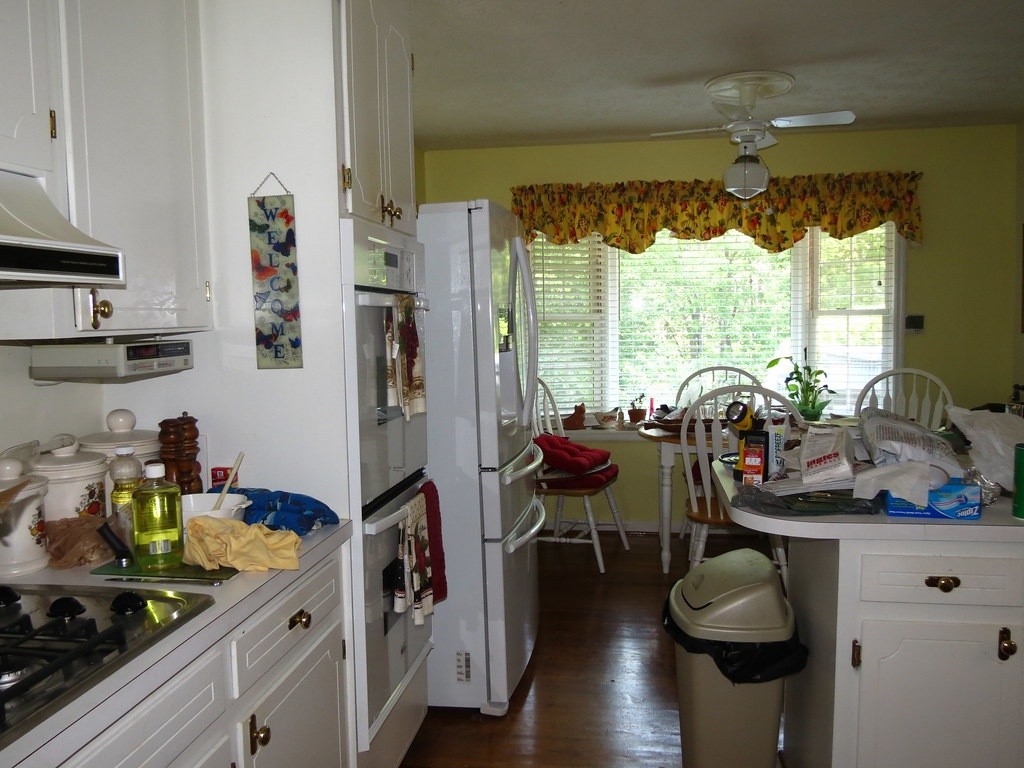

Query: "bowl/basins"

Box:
178,493,253,538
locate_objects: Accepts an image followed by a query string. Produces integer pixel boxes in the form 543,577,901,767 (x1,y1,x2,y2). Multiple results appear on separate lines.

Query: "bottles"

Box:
132,458,184,570
108,447,142,515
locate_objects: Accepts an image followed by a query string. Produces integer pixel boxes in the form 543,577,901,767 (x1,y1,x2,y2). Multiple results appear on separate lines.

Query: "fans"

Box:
650,72,857,152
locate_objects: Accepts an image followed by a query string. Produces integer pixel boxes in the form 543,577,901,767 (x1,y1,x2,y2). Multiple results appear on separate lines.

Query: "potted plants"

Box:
767,347,840,421
628,393,648,423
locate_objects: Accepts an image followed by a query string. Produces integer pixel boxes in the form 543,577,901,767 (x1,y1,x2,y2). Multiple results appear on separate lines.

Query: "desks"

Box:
638,425,741,573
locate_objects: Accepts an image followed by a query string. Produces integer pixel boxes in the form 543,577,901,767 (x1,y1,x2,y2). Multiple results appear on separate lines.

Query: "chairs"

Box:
854,367,954,430
681,385,806,591
532,377,631,574
677,366,769,561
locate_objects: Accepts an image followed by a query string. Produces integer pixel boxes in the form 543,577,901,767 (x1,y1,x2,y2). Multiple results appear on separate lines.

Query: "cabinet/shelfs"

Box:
56,546,351,768
0,0,56,178
850,554,1024,768
58,0,214,338
337,0,419,238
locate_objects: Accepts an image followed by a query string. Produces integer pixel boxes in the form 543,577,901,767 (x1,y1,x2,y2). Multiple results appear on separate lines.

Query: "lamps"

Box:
723,142,769,201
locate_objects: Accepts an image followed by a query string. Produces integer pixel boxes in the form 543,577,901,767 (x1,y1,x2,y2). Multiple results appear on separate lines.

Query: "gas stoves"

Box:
0,580,216,753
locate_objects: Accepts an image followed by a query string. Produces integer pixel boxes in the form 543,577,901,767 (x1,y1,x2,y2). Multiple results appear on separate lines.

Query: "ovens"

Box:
337,216,435,755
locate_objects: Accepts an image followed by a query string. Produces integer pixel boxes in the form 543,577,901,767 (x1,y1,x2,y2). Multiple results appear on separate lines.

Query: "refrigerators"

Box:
415,199,547,716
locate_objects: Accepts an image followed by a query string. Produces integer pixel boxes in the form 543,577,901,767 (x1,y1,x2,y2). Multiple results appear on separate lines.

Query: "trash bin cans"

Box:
663,548,795,767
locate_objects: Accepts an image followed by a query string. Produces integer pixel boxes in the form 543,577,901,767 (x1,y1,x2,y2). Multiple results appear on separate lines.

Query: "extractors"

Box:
0,171,127,291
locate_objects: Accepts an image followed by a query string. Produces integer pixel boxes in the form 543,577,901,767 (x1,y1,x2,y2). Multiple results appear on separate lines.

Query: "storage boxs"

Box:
743,444,765,487
211,466,239,488
886,478,981,520
